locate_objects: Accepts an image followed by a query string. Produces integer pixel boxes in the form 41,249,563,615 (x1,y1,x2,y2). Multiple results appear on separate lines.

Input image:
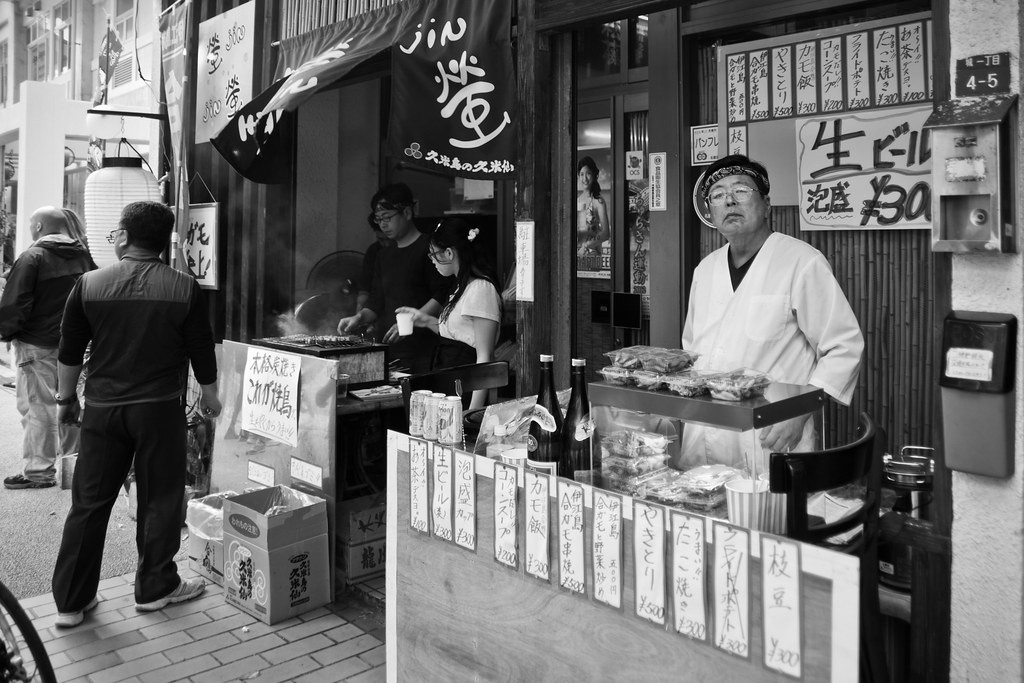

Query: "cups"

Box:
501,449,527,468
724,472,788,535
396,312,414,336
336,375,350,397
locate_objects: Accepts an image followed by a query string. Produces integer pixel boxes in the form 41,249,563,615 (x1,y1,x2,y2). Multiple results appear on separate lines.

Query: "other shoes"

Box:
4,473,56,488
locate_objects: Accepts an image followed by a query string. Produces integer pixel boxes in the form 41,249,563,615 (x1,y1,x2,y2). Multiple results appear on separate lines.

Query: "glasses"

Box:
705,185,763,206
373,211,401,224
109,227,127,237
426,250,442,261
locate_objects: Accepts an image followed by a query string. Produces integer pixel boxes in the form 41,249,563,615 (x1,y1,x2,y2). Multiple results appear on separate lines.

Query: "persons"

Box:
336,185,507,433
50,202,222,626
577,156,610,257
0,206,99,490
677,154,865,475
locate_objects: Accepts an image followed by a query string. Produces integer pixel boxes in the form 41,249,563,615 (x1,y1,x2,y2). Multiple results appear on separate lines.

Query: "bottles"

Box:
528,355,602,487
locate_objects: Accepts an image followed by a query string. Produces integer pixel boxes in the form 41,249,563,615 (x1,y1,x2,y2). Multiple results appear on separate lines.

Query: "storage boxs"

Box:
186,490,252,586
334,478,388,585
224,484,330,626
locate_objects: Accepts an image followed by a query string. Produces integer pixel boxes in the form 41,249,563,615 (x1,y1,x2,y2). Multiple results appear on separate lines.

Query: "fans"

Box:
307,250,369,334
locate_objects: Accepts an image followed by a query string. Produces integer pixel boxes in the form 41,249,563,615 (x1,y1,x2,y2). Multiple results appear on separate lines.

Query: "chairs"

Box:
398,359,509,435
766,410,889,682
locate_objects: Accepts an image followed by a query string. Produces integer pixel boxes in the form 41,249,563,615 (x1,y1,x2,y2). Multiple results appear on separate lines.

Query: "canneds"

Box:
408,391,463,447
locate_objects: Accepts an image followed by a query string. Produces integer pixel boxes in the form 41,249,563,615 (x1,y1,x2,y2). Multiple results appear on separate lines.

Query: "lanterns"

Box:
85,141,160,268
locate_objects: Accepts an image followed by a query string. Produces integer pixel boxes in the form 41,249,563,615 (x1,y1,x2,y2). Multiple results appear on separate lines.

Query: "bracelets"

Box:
54,393,78,405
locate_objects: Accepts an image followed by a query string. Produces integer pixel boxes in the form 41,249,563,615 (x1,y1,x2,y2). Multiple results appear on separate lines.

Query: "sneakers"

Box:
54,595,99,626
134,576,206,611
